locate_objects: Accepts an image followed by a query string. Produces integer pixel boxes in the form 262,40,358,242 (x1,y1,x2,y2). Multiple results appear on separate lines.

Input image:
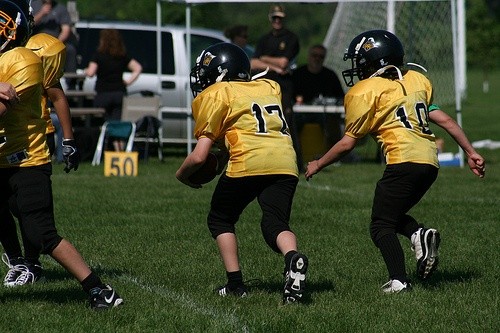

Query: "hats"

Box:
269,5,286,18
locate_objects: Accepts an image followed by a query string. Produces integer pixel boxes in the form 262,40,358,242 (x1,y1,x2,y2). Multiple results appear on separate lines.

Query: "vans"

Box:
50,20,256,148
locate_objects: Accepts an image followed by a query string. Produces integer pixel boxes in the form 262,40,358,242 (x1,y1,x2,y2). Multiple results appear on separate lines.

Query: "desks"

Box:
292,105,345,113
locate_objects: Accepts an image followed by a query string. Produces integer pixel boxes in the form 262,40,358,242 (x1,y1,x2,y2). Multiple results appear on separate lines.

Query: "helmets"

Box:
195,42,251,91
9,0,32,22
0,0,28,52
348,30,404,81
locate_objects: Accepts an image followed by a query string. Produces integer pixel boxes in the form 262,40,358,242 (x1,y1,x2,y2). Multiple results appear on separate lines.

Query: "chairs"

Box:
92,91,164,166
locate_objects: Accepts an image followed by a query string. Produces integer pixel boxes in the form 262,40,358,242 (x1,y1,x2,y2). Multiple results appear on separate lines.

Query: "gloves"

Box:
61,139,79,173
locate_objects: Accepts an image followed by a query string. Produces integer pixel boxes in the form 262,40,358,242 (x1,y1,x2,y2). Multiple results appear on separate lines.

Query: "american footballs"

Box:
189,153,219,184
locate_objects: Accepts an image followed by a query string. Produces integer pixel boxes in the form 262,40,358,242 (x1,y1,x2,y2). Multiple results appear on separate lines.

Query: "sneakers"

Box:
2,253,24,286
14,263,43,287
215,284,248,298
381,279,413,293
89,284,123,310
281,253,309,305
410,228,441,280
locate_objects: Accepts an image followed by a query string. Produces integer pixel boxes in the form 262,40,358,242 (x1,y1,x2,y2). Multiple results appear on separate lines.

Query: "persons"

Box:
306,30,485,296
0,0,388,309
175,43,309,306
